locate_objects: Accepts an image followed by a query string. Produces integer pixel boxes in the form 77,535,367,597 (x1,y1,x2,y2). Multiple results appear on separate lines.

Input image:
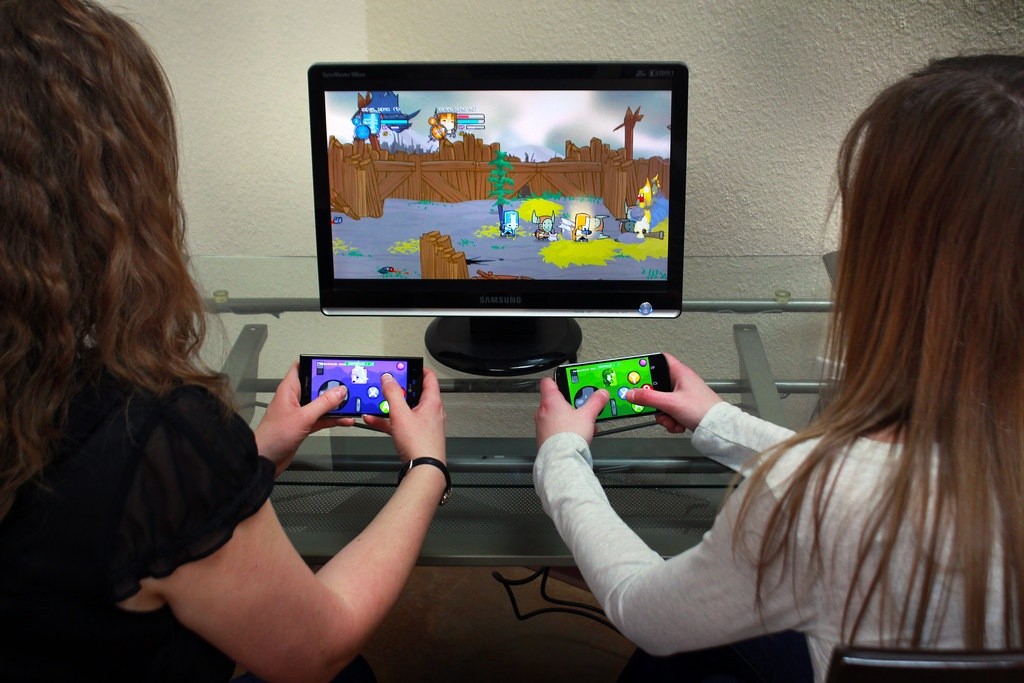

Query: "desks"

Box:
188,252,854,567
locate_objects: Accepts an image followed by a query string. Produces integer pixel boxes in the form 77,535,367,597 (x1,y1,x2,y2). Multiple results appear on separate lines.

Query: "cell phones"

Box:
299,354,423,417
555,352,673,423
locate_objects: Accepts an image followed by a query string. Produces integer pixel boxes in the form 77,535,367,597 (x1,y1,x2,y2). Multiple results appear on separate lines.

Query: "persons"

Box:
531,58,1024,683
0,0,454,683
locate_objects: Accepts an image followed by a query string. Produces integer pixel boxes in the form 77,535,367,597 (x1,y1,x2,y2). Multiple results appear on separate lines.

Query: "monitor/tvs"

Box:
308,61,688,377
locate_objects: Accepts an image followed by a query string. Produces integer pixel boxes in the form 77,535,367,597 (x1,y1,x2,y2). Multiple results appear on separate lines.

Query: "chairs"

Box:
825,646,1024,682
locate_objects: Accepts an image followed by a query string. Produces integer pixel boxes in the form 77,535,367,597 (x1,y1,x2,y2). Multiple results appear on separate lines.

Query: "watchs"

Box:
397,457,452,507
